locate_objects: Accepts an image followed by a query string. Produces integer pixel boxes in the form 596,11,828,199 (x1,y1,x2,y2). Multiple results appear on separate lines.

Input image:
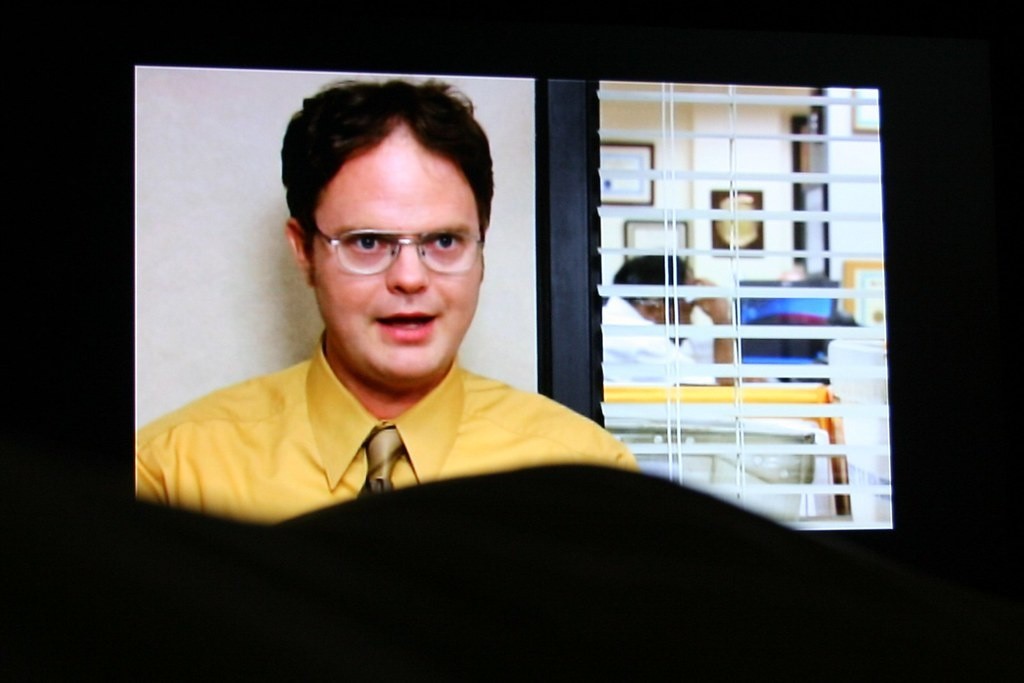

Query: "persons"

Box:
599,252,736,387
136,81,640,529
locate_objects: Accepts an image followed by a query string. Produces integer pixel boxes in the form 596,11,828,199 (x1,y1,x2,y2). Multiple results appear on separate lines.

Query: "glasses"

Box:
309,215,486,276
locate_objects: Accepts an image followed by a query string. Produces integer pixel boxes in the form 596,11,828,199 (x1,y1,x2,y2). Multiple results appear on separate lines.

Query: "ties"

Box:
356,426,405,496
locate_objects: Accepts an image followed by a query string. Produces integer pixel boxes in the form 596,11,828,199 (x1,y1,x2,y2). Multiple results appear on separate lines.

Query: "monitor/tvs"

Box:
743,282,840,366
